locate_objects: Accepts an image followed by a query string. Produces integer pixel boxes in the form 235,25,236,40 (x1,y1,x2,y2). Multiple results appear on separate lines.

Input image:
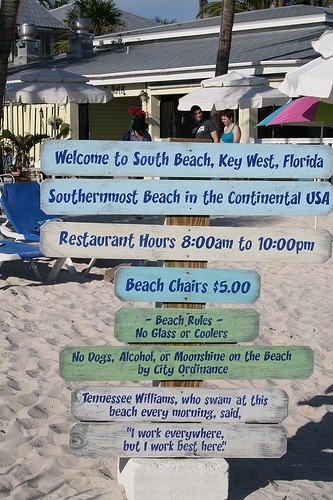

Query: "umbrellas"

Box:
176,70,289,110
4,65,113,104
276,28,333,102
253,96,333,144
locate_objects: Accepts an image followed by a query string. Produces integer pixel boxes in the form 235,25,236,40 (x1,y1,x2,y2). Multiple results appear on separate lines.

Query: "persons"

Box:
189,105,218,143
122,116,152,179
221,109,241,143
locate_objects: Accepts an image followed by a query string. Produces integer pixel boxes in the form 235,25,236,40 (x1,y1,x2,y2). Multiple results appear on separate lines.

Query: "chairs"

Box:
0,182,97,285
